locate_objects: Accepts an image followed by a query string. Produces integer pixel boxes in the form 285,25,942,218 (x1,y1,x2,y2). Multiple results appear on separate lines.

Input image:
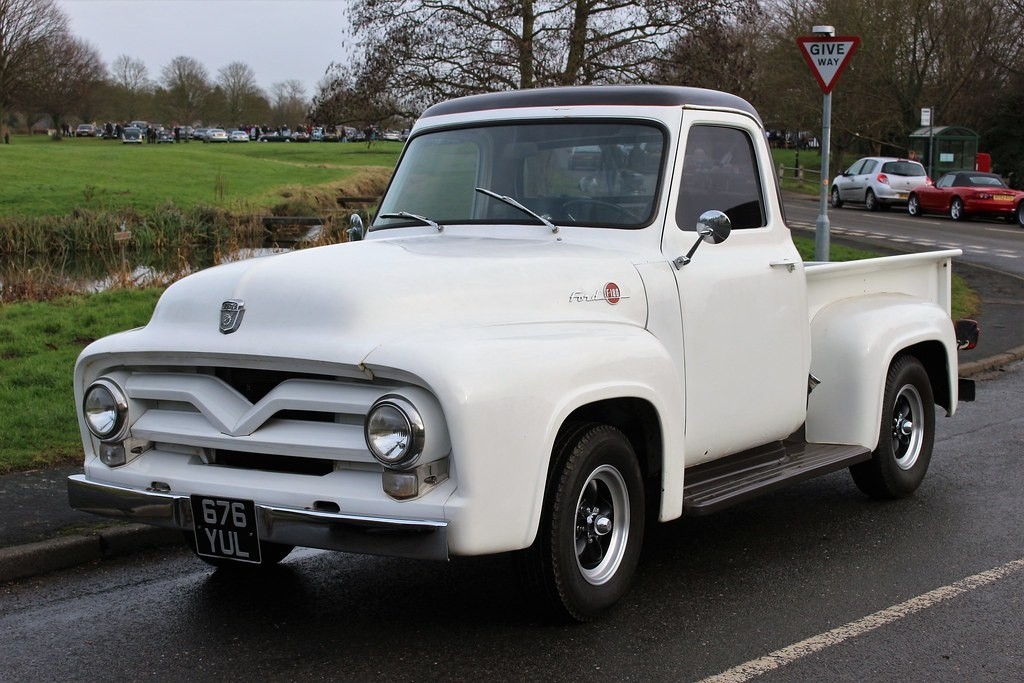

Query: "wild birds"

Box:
116,222,126,232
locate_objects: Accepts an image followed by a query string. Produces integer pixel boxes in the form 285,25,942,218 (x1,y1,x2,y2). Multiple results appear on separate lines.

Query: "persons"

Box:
576,146,739,197
0,119,387,151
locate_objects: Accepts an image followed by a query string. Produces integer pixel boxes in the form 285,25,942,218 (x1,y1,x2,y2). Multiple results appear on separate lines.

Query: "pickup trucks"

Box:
68,84,982,622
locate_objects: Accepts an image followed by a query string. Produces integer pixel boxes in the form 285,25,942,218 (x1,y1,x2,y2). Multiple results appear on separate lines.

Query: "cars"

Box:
122,127,143,144
101,123,118,138
229,131,249,143
148,124,164,134
203,129,228,143
75,124,95,137
569,145,603,170
194,128,208,140
907,170,1022,223
172,126,193,139
1013,192,1024,228
158,130,173,144
131,121,148,140
248,126,410,141
831,157,935,212
